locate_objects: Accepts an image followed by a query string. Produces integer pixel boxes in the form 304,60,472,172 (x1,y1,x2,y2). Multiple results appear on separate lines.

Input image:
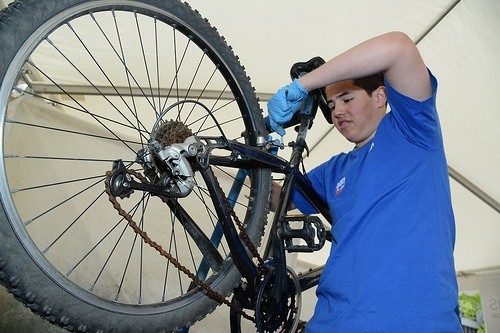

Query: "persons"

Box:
244,32,464,333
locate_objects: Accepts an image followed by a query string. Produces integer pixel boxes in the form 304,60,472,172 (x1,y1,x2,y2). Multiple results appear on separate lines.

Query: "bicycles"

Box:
0,0,332,333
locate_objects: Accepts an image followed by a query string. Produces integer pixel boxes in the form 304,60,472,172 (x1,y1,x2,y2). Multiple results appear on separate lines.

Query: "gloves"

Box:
267,78,308,136
244,135,280,176
